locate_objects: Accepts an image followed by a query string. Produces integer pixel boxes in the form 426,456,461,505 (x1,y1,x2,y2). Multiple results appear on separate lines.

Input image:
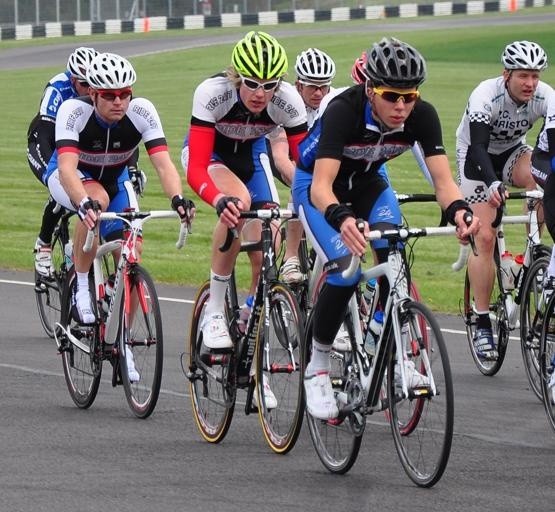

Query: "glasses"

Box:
95,88,132,101
371,81,421,104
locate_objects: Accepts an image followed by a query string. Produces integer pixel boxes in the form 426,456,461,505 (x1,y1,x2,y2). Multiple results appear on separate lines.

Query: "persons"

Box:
179,29,309,410
266,44,336,285
454,39,555,361
530,90,555,305
290,35,483,421
42,51,197,384
26,45,148,282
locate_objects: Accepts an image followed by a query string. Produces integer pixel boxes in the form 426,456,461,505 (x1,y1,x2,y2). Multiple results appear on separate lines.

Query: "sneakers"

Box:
125,344,140,382
281,256,303,285
71,292,97,326
384,359,431,389
304,363,340,420
200,306,234,351
473,323,499,361
251,373,278,409
32,240,56,280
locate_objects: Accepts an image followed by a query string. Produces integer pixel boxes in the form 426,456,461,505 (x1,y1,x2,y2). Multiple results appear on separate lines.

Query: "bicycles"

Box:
52,208,200,421
32,169,145,343
520,257,552,402
538,281,555,432
460,189,552,392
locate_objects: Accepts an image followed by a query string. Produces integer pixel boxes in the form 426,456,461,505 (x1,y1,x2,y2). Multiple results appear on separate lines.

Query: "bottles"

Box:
364,277,377,305
100,272,114,313
502,249,513,288
505,253,524,291
63,239,74,270
363,309,385,355
236,293,253,334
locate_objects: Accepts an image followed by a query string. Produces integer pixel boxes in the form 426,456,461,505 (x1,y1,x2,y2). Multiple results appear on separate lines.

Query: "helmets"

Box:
231,30,426,89
66,47,137,90
501,40,548,72
